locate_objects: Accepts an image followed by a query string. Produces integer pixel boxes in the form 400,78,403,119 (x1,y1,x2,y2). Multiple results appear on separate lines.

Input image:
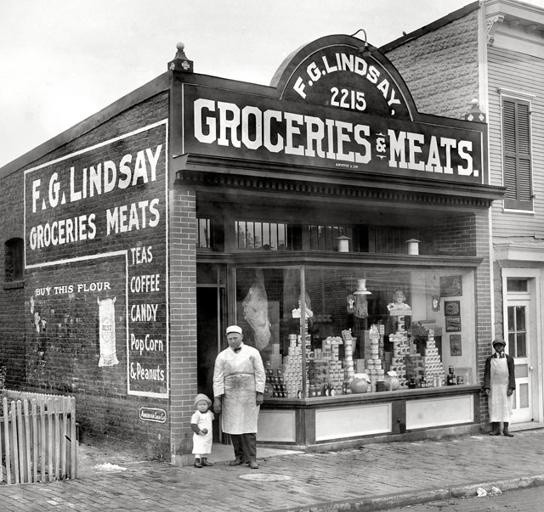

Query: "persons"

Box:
190,393,222,469
212,324,266,469
388,289,413,317
483,338,516,438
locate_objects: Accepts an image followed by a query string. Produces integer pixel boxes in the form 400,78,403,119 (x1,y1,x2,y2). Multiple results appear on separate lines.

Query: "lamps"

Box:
354,277,373,296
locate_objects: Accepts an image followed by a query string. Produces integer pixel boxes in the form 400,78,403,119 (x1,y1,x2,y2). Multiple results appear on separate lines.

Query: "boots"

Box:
194,458,212,467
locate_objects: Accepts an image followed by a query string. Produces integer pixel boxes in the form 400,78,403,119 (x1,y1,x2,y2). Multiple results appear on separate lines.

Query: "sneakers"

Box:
490,431,500,435
230,460,243,465
249,461,258,469
503,432,513,437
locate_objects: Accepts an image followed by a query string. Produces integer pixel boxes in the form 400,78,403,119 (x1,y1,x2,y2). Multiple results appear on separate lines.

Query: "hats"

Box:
226,325,242,334
194,394,212,407
493,339,506,347
